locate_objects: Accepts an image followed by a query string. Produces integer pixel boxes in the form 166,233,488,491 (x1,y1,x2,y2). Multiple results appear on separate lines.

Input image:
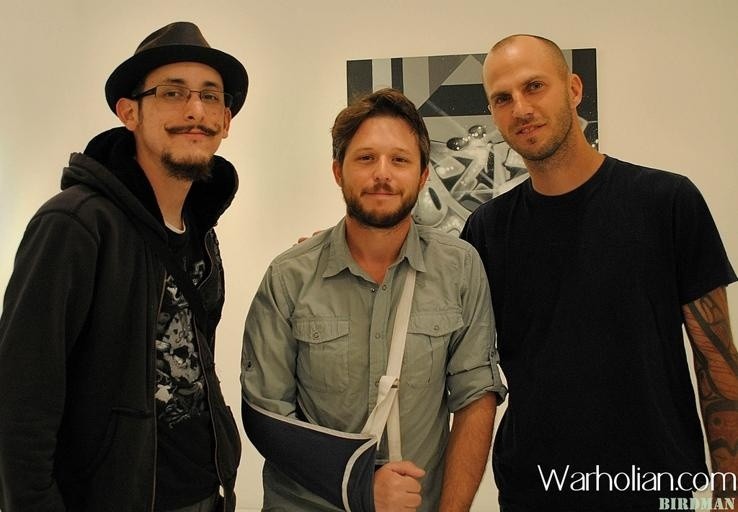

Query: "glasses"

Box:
130,85,233,111
447,125,486,151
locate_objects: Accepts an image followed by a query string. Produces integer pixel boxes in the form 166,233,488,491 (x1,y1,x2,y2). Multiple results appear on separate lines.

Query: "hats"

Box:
105,22,248,118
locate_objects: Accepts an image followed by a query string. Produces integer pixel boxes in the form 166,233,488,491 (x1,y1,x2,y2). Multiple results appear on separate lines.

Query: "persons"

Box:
461,34,737,511
239,87,507,512
0,22,248,511
